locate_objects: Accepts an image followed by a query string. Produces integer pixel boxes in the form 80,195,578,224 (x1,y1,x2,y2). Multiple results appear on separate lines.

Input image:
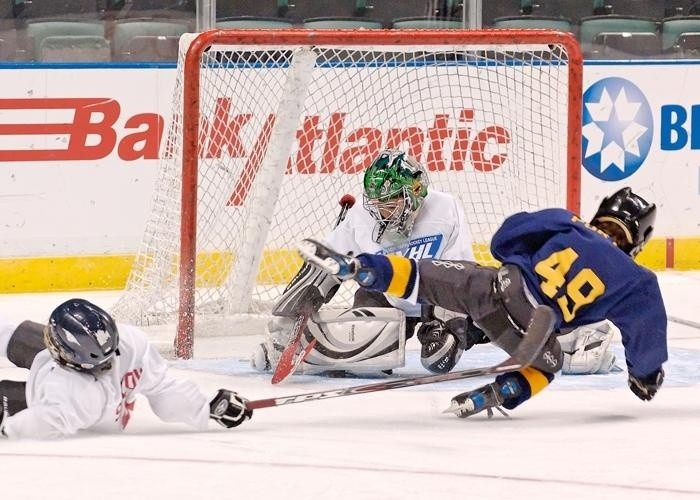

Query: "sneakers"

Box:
450,374,521,415
295,239,376,289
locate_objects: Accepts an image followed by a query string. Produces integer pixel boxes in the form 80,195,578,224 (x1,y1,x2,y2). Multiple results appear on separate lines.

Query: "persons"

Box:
248,142,619,378
0,297,253,441
293,184,670,421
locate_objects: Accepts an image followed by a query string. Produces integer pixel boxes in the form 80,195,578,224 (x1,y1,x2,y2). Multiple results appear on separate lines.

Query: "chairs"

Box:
0,0,700,63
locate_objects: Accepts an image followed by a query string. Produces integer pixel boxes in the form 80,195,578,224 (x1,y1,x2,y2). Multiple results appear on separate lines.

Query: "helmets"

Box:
41,297,120,370
363,148,431,232
590,186,658,259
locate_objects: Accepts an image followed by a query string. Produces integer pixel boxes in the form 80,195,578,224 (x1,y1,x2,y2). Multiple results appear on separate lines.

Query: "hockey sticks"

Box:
271,193,356,384
244,304,556,411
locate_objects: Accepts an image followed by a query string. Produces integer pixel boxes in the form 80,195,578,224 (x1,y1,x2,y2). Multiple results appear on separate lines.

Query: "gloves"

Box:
206,387,253,432
627,370,665,406
